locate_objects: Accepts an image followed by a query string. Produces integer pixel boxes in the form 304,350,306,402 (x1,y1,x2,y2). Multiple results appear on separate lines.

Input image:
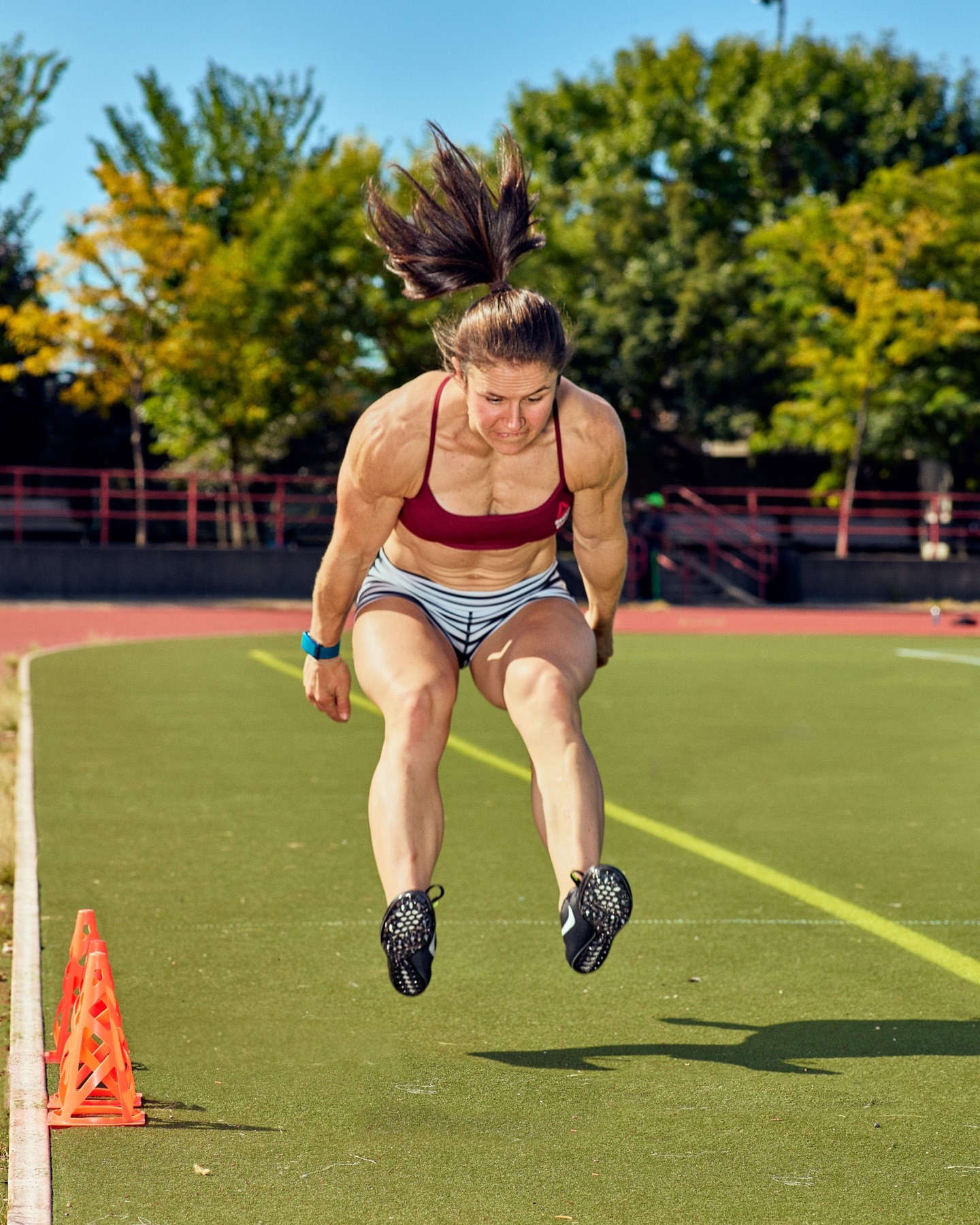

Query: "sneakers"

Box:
379,885,444,996
559,864,632,974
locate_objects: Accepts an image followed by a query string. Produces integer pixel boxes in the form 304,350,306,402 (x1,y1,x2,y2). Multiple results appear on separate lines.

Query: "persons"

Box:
302,118,631,996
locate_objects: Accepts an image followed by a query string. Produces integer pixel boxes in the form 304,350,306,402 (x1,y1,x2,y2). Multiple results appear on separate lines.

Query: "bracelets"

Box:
302,632,340,659
